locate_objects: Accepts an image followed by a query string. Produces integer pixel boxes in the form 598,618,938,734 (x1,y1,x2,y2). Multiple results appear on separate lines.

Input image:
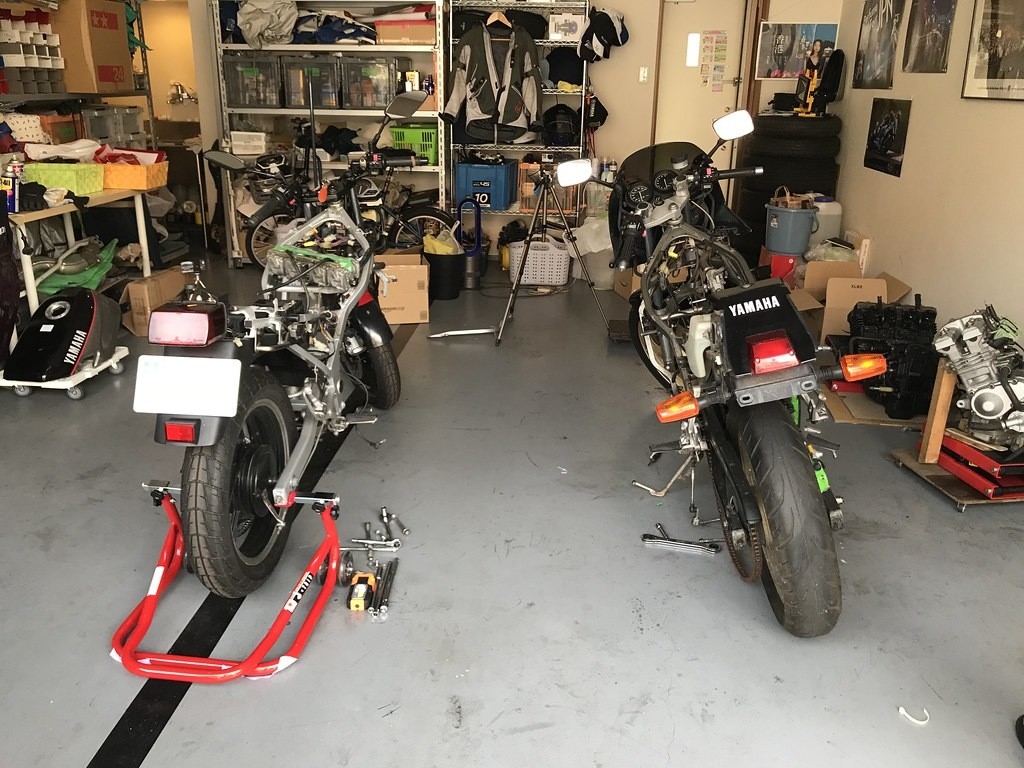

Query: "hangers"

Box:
485,4,513,41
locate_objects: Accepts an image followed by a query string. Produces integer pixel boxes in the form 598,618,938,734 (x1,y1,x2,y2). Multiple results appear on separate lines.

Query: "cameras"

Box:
542,153,553,163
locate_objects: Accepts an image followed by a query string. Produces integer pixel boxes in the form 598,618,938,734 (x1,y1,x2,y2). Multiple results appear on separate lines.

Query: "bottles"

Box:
0,154,25,215
427,75,434,95
405,69,421,92
591,156,617,180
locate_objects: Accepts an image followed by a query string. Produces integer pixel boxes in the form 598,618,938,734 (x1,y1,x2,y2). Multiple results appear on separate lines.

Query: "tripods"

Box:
495,163,609,346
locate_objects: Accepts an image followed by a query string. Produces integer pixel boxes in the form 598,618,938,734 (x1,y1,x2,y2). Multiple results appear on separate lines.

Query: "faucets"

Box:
166,81,182,104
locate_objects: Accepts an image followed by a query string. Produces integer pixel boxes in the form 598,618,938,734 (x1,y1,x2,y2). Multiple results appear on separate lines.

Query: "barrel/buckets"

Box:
801,193,842,248
421,245,490,298
765,203,820,256
587,250,613,291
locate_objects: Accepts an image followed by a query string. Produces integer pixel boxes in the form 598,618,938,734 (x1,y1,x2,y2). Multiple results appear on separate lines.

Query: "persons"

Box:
806,39,824,76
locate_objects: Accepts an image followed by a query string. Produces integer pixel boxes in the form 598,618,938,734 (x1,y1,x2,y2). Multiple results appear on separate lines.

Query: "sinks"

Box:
143,120,199,140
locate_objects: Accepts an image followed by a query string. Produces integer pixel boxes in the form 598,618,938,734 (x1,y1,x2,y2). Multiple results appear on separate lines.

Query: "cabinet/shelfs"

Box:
7,188,159,321
211,0,449,269
451,0,591,275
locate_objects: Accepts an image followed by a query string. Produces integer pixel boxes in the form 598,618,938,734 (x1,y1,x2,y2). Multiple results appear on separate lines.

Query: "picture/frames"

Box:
755,22,840,81
960,0,1024,101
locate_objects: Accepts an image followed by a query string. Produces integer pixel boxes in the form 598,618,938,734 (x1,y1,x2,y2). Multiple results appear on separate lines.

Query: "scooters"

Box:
244,148,464,280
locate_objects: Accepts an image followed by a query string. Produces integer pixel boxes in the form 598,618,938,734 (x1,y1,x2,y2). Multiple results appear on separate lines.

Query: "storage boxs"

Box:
223,52,412,110
372,245,430,324
759,246,913,347
39,105,147,153
519,163,586,213
24,162,104,196
614,264,689,299
374,20,436,45
455,159,518,210
102,95,150,120
118,265,195,337
104,160,169,191
417,95,435,111
549,12,585,41
845,231,870,279
271,134,340,162
49,0,135,93
230,131,273,154
390,122,438,167
238,219,263,257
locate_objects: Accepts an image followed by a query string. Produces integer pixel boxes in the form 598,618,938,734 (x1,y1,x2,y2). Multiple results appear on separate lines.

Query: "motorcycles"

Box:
131,82,428,605
556,107,889,636
